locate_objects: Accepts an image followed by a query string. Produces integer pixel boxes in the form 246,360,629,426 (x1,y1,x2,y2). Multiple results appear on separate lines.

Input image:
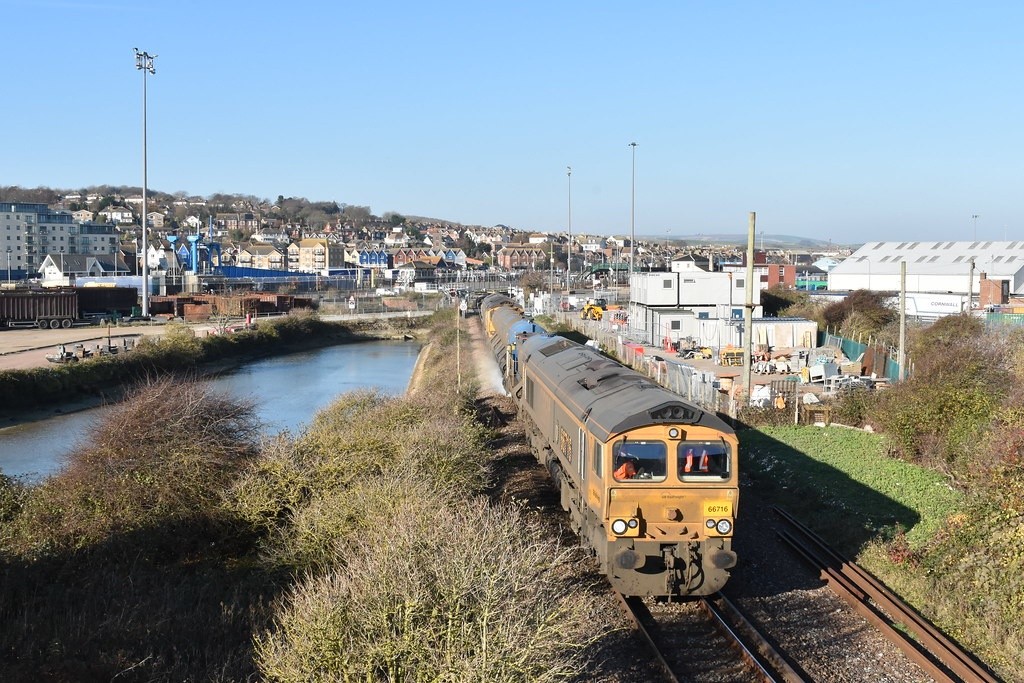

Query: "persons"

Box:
614,456,640,479
681,443,713,475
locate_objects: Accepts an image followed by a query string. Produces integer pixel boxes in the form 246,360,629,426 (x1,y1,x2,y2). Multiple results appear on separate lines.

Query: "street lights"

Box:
566,143,636,294
112,250,120,276
6,248,13,284
59,250,66,276
134,47,158,317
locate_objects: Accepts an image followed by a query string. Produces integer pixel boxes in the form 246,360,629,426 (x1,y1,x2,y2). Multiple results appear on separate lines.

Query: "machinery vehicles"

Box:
580,298,608,321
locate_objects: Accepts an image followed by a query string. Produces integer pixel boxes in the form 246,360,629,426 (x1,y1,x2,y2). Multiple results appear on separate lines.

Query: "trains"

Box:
0,284,138,329
479,294,739,596
